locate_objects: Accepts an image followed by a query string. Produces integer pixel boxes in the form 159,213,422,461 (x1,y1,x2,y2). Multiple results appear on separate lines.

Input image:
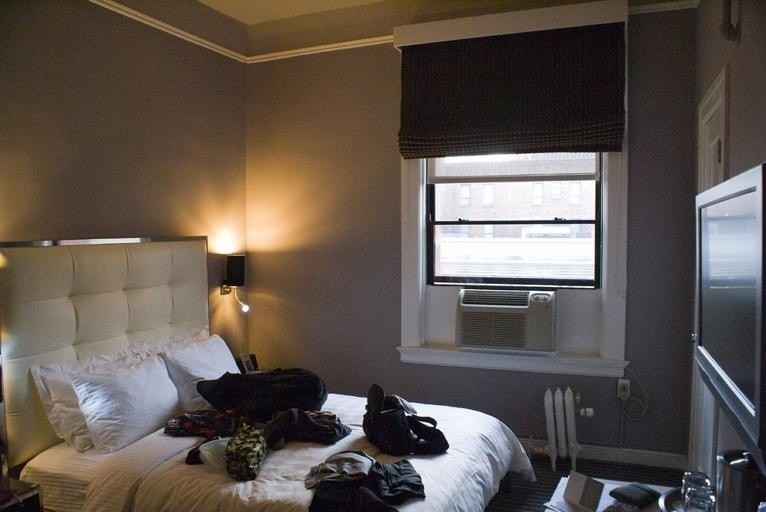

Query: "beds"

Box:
1,234,540,509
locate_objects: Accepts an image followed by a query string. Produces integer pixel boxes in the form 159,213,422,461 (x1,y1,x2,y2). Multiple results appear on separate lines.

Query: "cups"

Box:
682,468,716,512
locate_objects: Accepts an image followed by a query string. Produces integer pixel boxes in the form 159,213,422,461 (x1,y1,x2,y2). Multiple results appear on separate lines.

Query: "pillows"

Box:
28,325,240,461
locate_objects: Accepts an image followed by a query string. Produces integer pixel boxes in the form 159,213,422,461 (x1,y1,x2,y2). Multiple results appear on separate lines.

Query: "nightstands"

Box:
2,469,44,511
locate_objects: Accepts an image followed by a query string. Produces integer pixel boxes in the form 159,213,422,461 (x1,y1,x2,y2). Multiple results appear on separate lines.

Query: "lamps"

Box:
218,250,254,313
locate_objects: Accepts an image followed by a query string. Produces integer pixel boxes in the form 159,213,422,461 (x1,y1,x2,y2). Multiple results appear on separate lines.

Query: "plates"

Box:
658,486,682,511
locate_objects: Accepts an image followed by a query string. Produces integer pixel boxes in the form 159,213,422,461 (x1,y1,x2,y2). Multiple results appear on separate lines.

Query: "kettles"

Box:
715,449,762,511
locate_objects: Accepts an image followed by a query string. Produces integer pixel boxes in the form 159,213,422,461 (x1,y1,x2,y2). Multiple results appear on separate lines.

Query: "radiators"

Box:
534,386,595,476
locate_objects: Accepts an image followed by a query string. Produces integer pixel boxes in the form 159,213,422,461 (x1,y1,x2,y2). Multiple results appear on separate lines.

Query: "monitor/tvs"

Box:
695,163,766,447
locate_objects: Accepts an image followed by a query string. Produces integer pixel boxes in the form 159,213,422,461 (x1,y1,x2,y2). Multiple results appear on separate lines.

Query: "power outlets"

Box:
617,379,632,401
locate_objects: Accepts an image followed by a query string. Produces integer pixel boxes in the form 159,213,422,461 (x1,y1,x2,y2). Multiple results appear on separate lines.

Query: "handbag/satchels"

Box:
363,384,449,457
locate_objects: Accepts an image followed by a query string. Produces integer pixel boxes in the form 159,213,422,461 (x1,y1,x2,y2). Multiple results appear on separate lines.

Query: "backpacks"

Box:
185,366,327,466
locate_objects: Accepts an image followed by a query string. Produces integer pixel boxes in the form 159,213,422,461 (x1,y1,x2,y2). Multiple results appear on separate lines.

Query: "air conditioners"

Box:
453,286,558,355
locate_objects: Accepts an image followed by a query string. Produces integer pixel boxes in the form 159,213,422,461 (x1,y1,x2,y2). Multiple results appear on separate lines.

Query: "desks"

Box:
543,468,717,511
